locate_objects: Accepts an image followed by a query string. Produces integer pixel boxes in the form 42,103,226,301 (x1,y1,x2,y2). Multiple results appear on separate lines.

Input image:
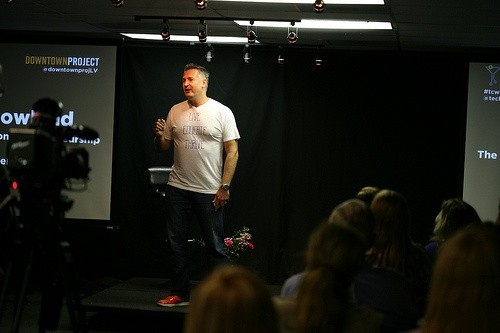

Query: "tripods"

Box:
0,190,87,333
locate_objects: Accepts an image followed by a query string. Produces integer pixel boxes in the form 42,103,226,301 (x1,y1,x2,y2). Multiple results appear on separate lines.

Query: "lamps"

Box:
278,45,285,65
196,22,210,42
246,24,257,43
242,48,253,64
315,44,324,66
286,28,298,44
312,0,326,14
204,44,214,63
195,1,208,11
160,20,171,42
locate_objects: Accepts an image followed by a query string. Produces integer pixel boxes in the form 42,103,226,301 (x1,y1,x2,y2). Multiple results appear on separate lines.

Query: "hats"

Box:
30,98,62,115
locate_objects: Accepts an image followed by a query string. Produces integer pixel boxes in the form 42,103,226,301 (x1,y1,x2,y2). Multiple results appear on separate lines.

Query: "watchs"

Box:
219,184,230,191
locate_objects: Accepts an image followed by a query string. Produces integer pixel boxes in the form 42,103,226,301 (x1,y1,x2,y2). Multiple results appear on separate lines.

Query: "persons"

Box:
153,64,242,307
184,185,500,333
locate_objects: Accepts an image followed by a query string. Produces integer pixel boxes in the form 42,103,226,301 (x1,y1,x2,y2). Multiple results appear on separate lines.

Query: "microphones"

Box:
152,111,167,144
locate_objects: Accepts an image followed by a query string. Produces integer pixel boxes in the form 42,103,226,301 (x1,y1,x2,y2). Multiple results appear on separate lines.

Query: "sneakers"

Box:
157,294,189,307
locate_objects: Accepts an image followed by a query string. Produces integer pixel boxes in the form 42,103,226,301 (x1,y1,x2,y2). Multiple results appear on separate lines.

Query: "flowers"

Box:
222,226,255,265
